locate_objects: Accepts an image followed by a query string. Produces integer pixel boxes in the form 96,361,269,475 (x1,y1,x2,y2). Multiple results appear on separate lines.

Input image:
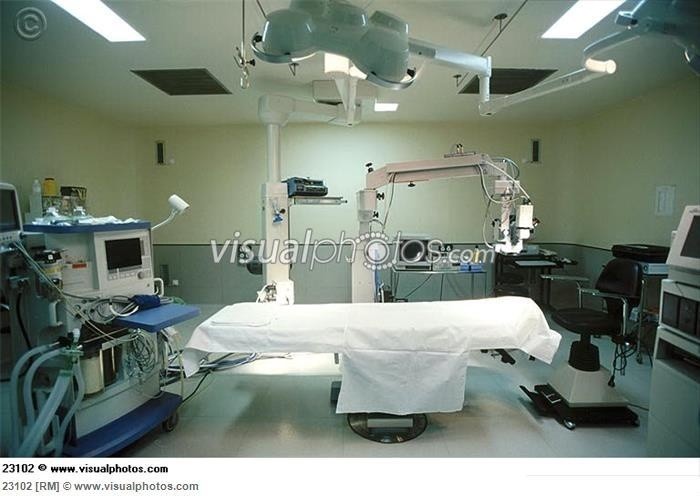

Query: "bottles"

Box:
29,176,60,220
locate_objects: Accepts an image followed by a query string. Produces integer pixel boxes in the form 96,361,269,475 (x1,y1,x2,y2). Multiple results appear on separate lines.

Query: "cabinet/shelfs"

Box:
643,280,700,457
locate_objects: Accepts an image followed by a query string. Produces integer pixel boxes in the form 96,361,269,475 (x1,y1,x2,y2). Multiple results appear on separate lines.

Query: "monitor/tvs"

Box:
89,229,153,290
665,205,700,275
396,237,431,266
0,182,23,254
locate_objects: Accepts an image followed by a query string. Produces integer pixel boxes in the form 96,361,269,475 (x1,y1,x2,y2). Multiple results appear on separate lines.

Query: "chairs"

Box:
519,255,643,432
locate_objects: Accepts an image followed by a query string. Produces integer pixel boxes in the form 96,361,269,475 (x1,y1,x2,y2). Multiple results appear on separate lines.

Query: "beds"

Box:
192,294,548,445
